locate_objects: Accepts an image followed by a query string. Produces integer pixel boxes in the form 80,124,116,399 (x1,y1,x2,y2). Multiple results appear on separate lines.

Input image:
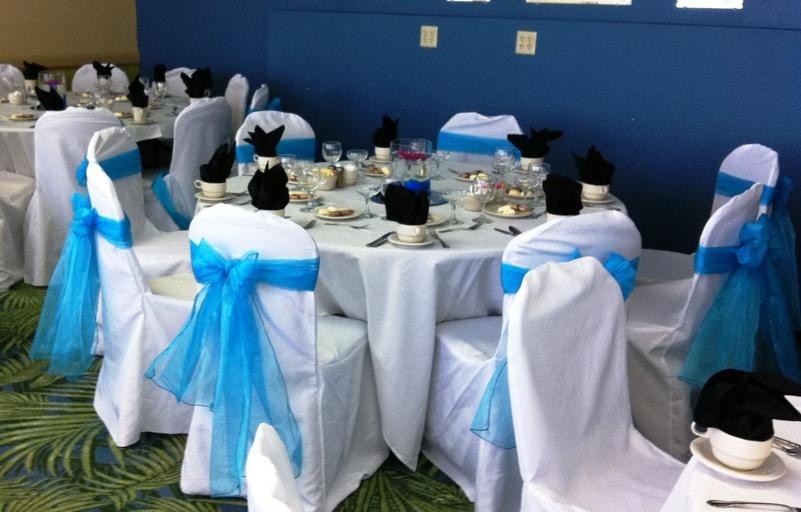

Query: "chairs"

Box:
247,84,281,114
436,111,520,161
142,202,388,512
142,99,231,232
0,64,27,101
510,255,684,512
1,168,33,293
636,142,777,288
226,74,249,132
30,127,201,449
234,110,318,176
627,187,776,460
71,63,129,93
422,212,640,512
164,68,196,98
25,107,126,287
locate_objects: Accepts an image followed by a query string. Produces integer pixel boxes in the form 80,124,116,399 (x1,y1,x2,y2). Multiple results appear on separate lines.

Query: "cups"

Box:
8,93,24,105
262,209,285,217
24,79,37,97
691,419,774,472
375,146,391,161
388,138,432,182
194,179,227,197
579,180,611,200
395,222,426,243
253,154,279,172
457,171,495,181
132,106,148,122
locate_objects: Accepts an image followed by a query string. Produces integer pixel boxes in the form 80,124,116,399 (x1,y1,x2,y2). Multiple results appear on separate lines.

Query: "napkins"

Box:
374,116,399,149
248,160,290,209
692,366,797,441
507,128,563,158
32,87,66,112
180,69,211,96
385,182,428,226
199,142,234,182
127,77,149,108
153,65,167,82
23,62,42,81
542,174,584,215
242,125,285,158
90,62,113,80
575,146,614,186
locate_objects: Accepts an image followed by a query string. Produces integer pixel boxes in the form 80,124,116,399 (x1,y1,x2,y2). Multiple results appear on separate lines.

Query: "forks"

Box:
428,226,449,249
707,499,801,512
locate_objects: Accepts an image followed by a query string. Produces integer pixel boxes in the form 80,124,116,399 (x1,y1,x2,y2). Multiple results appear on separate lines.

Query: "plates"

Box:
688,434,789,482
194,191,233,202
484,198,535,218
127,119,153,125
369,155,392,163
314,204,363,220
387,233,434,247
256,210,313,228
9,117,36,121
581,198,616,204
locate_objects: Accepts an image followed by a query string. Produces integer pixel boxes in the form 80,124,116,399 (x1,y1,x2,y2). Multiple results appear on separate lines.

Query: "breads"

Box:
291,192,309,200
318,206,353,217
509,188,522,196
497,203,529,215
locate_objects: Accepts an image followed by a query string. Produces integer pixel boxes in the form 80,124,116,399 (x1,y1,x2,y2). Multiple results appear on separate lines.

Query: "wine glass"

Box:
138,77,168,105
495,146,515,183
527,163,550,209
322,141,343,169
346,149,369,183
279,153,321,213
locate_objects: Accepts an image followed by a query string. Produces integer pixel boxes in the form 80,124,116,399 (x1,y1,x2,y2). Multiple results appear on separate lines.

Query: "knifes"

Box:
494,226,522,236
367,232,396,247
